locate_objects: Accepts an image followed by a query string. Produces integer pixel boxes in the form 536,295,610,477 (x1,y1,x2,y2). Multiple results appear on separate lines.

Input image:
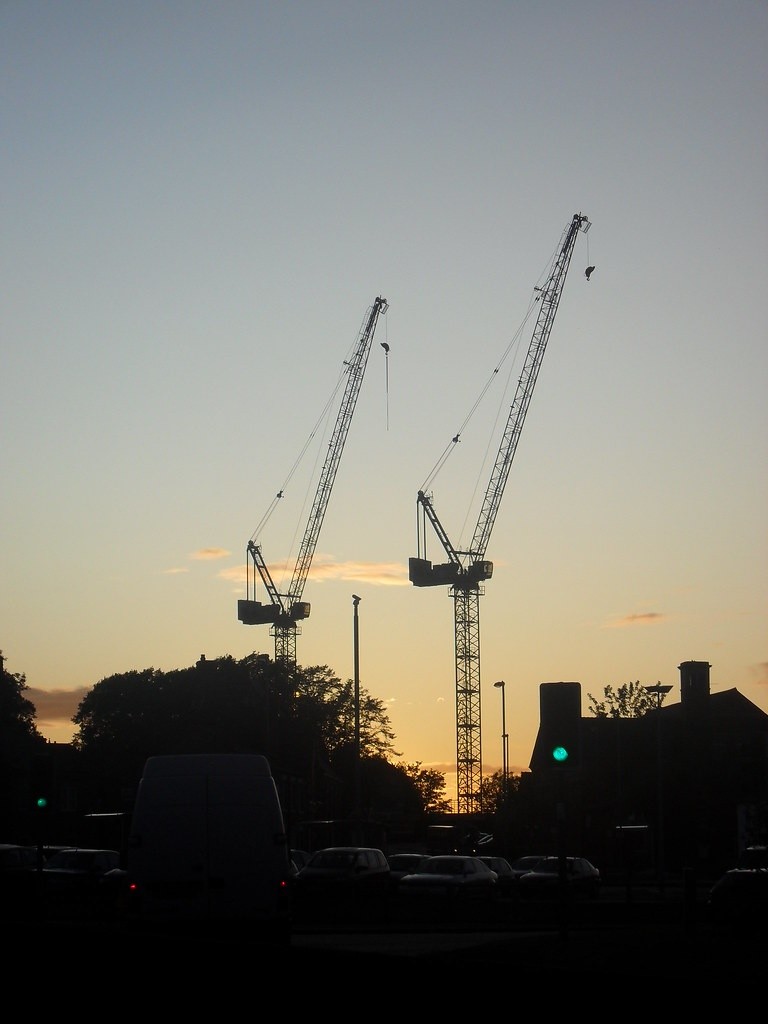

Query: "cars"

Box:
1,752,768,948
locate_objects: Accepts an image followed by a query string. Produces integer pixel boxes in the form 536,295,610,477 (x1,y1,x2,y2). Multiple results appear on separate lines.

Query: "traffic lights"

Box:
550,743,570,762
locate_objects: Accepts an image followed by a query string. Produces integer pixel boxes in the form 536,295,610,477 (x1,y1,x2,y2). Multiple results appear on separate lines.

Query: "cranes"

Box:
408,213,599,818
233,296,393,747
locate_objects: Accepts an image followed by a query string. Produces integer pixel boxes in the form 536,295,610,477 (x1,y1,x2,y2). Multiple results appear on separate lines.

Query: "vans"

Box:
122,750,295,945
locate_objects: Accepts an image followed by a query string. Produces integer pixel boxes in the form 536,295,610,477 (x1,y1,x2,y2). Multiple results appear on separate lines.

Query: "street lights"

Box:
493,680,509,810
352,594,362,748
